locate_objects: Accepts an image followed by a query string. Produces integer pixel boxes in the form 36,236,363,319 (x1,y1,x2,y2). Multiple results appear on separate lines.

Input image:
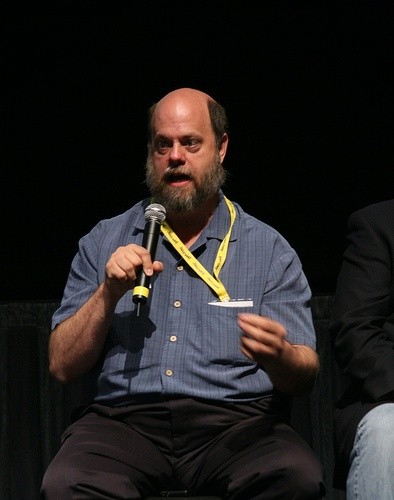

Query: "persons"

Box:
331,201,394,500
42,86,325,500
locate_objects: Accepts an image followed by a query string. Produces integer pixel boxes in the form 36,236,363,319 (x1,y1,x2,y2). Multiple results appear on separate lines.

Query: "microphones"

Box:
135,203,167,318
